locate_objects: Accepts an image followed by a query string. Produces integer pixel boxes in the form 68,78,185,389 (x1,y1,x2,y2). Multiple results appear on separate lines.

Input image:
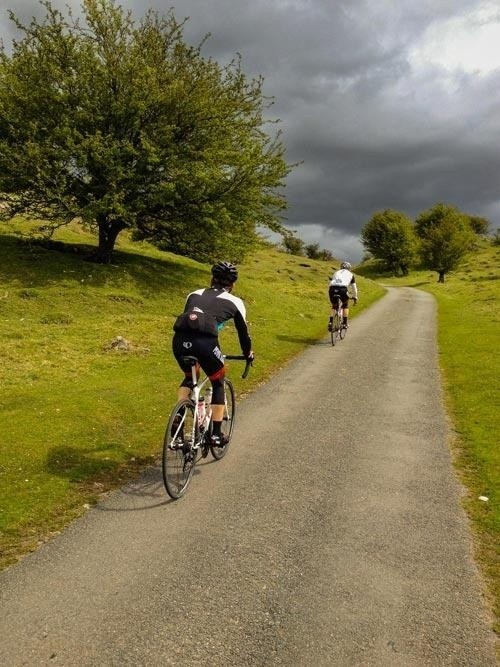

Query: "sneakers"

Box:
211,433,231,447
170,416,184,440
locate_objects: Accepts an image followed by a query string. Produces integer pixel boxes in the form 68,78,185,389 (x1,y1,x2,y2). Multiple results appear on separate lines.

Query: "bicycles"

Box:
162,354,254,499
328,295,358,346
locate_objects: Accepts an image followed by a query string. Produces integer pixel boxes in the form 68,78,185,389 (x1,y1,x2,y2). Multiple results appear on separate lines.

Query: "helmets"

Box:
340,262,351,269
211,261,238,281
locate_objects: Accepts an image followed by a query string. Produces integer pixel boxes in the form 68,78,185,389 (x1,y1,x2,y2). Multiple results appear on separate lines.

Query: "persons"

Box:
326,260,358,331
168,260,254,447
